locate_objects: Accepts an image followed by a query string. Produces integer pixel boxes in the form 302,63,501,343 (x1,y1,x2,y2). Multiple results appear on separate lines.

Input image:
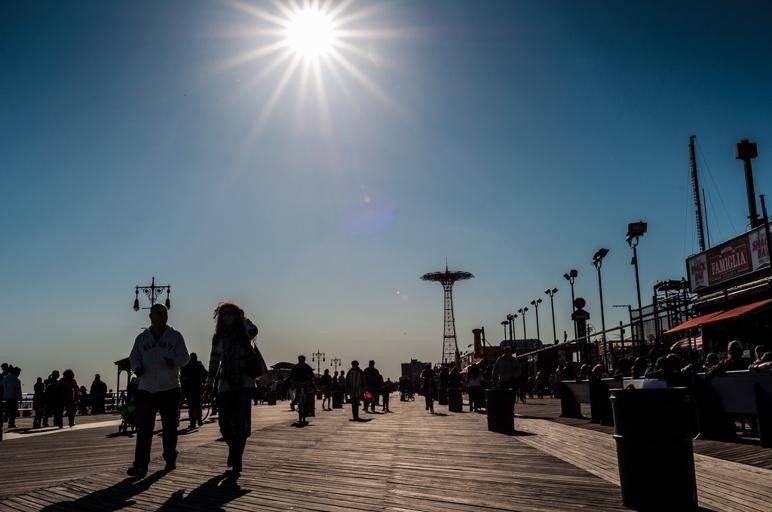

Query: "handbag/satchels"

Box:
248,345,268,379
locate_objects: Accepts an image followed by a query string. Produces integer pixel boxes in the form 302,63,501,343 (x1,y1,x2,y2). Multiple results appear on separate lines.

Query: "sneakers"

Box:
7,423,74,428
425,396,555,414
291,403,392,423
124,457,177,477
226,448,244,474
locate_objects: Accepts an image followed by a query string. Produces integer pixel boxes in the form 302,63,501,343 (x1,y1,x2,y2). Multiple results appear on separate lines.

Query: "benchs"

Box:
552,358,772,450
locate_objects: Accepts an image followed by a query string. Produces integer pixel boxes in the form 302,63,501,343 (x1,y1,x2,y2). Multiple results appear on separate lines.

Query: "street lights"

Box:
529,298,542,342
501,312,517,341
591,246,611,376
545,288,559,345
563,269,579,364
312,349,326,399
517,306,528,340
132,276,172,311
626,219,649,355
330,355,341,371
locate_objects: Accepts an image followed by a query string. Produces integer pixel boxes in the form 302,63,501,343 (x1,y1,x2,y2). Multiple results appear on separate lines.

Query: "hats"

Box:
727,339,743,351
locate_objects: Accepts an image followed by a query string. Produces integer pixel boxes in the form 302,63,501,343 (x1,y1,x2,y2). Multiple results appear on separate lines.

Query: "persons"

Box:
0,362,136,432
252,342,771,434
127,303,190,476
208,303,258,474
180,353,208,428
210,365,220,416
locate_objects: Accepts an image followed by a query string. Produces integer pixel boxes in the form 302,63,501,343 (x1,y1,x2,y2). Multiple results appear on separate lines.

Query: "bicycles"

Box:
405,394,415,402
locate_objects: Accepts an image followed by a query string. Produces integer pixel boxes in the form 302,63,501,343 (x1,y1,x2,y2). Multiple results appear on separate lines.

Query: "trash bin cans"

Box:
446,388,463,412
332,390,344,408
609,386,699,512
485,388,517,434
317,390,322,400
437,388,447,405
303,392,315,417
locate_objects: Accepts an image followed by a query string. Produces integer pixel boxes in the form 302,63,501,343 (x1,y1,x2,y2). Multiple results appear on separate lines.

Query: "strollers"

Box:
117,391,137,432
